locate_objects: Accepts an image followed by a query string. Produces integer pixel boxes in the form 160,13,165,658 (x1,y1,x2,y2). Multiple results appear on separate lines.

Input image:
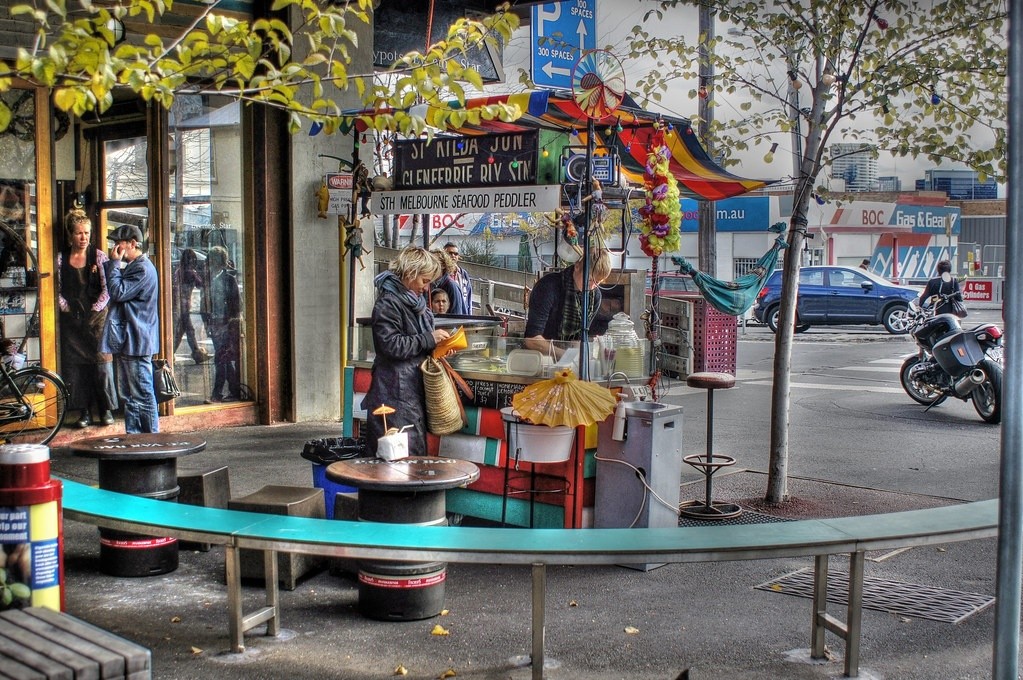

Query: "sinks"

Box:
625,401,667,411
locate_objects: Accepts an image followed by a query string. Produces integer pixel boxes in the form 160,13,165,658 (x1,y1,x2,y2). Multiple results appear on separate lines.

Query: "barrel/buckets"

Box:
99,457,179,577
358,488,449,622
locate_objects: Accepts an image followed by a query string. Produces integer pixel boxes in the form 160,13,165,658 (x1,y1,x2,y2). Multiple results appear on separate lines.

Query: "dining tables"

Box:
68,434,206,578
326,456,481,620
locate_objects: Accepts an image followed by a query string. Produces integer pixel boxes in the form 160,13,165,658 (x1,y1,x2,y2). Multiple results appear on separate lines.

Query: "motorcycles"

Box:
900,272,1002,425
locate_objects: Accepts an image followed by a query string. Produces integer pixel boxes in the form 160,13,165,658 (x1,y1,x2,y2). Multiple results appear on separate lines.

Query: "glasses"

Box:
449,252,459,255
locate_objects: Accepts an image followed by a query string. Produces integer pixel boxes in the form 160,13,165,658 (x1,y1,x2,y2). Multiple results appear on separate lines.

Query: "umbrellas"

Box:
511,369,618,430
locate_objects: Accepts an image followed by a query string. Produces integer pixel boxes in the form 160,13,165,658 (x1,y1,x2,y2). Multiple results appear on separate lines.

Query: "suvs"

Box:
753,266,925,334
645,269,701,298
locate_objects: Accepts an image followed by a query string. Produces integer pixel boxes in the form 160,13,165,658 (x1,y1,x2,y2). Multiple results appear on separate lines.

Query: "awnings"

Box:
309,89,783,380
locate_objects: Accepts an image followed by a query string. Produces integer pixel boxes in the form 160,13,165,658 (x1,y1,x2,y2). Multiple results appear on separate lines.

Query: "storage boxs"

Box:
664,295,738,378
933,330,985,376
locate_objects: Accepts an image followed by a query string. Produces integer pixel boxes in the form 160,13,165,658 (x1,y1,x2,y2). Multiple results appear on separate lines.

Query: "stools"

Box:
679,371,743,519
178,465,230,551
228,485,324,591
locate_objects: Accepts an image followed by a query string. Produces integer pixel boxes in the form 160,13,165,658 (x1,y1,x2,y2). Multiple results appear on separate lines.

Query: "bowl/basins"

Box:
501,406,575,464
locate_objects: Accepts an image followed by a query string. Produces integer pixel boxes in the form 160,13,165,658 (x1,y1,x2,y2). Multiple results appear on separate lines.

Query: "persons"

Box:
423,242,472,314
173,245,243,402
853,259,870,284
98,225,160,434
919,259,967,315
360,243,456,456
57,209,119,428
524,246,612,361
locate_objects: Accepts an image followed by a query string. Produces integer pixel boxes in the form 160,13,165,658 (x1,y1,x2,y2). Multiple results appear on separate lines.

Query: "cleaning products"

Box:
611,392,629,441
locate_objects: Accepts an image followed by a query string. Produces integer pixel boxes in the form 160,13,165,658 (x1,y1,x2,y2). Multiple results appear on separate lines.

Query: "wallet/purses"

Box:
431,324,468,362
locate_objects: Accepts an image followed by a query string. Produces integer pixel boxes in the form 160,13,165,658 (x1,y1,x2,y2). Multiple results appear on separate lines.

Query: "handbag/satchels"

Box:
420,357,464,436
218,329,240,363
950,297,968,318
151,358,181,403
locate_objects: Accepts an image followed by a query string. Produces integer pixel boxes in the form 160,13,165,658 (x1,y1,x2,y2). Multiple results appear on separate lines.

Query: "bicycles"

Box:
0,336,70,446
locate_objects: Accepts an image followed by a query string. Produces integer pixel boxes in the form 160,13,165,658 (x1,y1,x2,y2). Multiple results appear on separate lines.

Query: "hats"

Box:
107,225,143,243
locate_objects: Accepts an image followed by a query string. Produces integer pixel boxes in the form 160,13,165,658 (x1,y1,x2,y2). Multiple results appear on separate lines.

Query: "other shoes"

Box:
191,348,215,363
98,409,114,425
74,406,94,428
203,396,223,404
222,394,240,401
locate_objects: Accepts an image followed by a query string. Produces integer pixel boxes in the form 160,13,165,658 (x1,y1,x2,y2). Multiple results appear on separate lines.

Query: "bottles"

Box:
602,312,643,378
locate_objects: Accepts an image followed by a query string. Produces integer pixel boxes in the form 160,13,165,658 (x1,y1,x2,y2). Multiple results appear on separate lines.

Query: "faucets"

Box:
607,371,631,390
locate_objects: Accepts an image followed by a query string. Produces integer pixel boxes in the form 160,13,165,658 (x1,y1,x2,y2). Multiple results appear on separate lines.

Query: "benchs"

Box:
0,606,151,680
50,474,1000,678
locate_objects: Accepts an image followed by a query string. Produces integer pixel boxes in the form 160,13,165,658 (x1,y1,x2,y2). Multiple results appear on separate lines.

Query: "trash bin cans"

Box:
303,433,377,523
